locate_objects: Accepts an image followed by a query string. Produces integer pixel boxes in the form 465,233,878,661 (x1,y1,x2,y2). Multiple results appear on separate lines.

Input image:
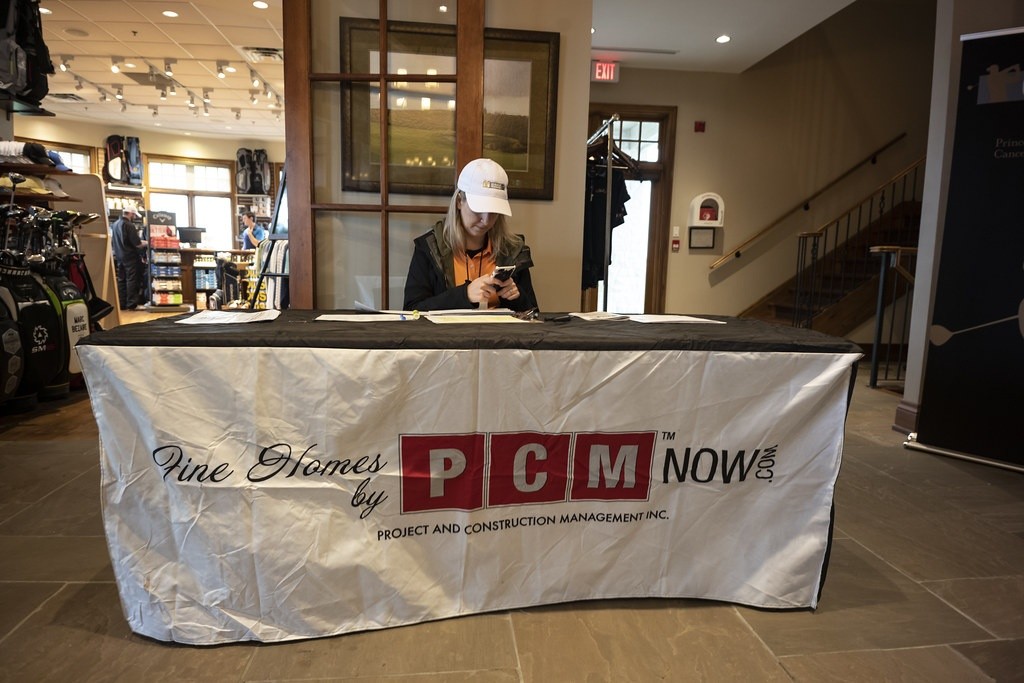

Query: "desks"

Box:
76,307,865,642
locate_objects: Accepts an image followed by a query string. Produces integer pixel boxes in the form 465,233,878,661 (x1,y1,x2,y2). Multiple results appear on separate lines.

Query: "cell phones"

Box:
490,265,516,293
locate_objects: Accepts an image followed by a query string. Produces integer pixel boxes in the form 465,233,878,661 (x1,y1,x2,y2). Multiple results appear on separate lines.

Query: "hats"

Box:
122,205,142,219
457,158,513,217
0,141,74,198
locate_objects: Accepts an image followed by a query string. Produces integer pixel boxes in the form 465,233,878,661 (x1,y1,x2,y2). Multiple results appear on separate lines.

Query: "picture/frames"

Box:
339,15,561,201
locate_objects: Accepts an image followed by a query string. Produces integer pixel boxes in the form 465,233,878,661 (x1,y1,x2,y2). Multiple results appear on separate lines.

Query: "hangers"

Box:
586,124,645,185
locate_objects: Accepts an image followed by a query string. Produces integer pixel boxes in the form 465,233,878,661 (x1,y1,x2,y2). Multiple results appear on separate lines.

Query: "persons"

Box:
237,210,264,261
404,157,539,319
109,205,151,310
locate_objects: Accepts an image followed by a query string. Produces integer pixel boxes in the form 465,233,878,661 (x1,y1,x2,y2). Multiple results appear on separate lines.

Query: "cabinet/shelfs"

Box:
106,179,272,310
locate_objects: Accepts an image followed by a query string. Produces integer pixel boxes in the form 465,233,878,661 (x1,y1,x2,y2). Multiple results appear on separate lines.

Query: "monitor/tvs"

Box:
178,226,202,243
